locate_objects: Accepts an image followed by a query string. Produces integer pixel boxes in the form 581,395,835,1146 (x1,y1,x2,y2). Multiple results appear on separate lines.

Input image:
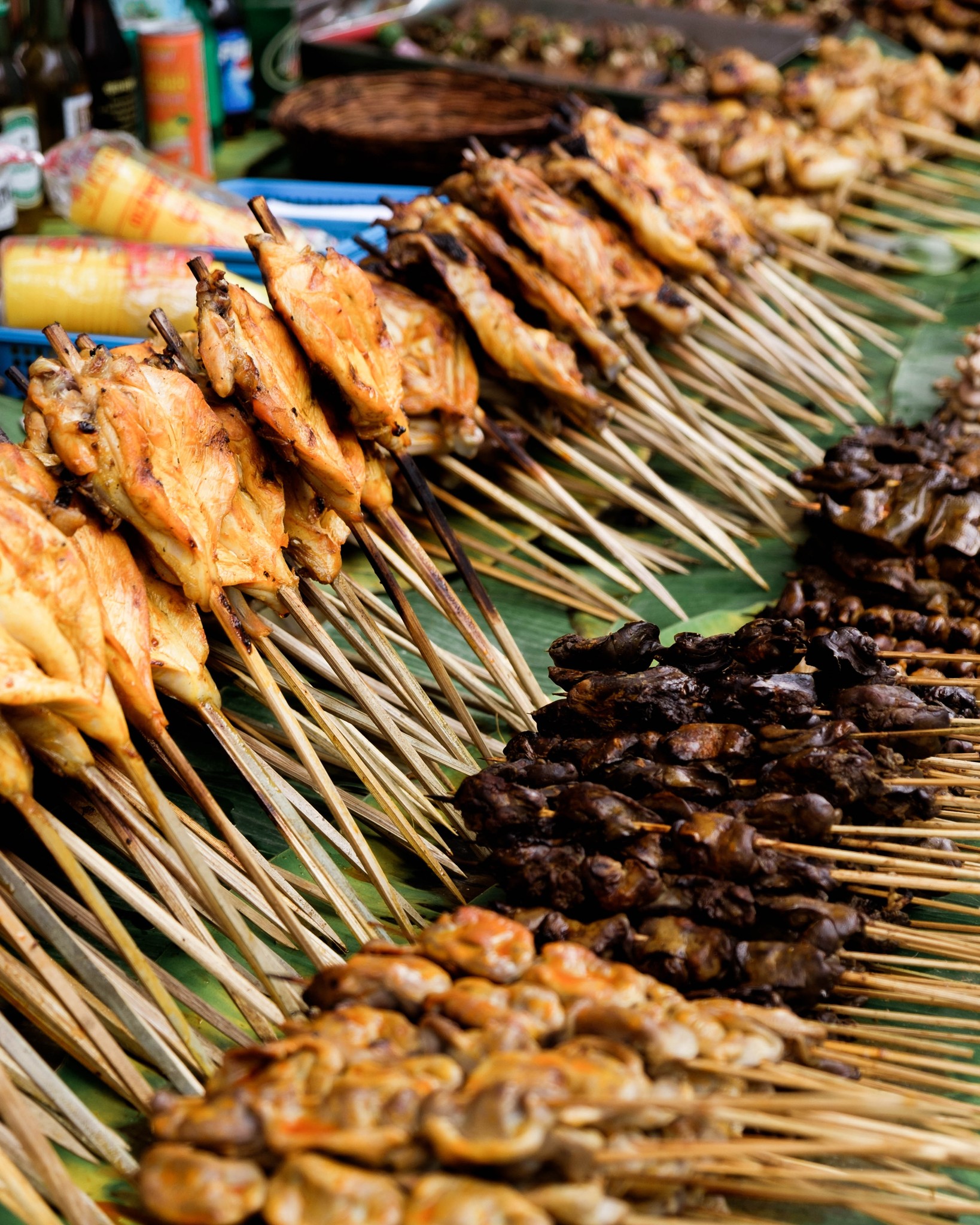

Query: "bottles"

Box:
1,54,46,214
214,10,255,138
63,82,93,139
68,0,147,146
